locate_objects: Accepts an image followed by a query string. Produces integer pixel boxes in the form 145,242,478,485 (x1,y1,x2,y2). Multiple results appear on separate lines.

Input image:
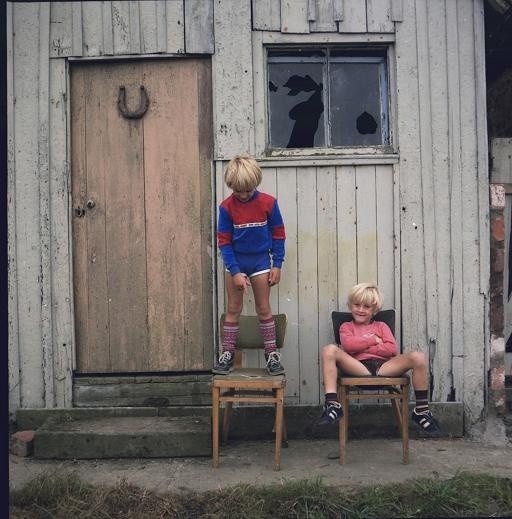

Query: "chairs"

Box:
211,314,287,470
330,309,410,465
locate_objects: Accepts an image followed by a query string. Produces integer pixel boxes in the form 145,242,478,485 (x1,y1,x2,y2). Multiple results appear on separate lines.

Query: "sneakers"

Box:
263,349,286,376
411,407,441,434
211,350,235,375
317,400,344,428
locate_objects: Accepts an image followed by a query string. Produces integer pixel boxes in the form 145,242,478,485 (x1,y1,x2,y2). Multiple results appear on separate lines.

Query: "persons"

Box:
318,282,441,436
211,153,286,376
